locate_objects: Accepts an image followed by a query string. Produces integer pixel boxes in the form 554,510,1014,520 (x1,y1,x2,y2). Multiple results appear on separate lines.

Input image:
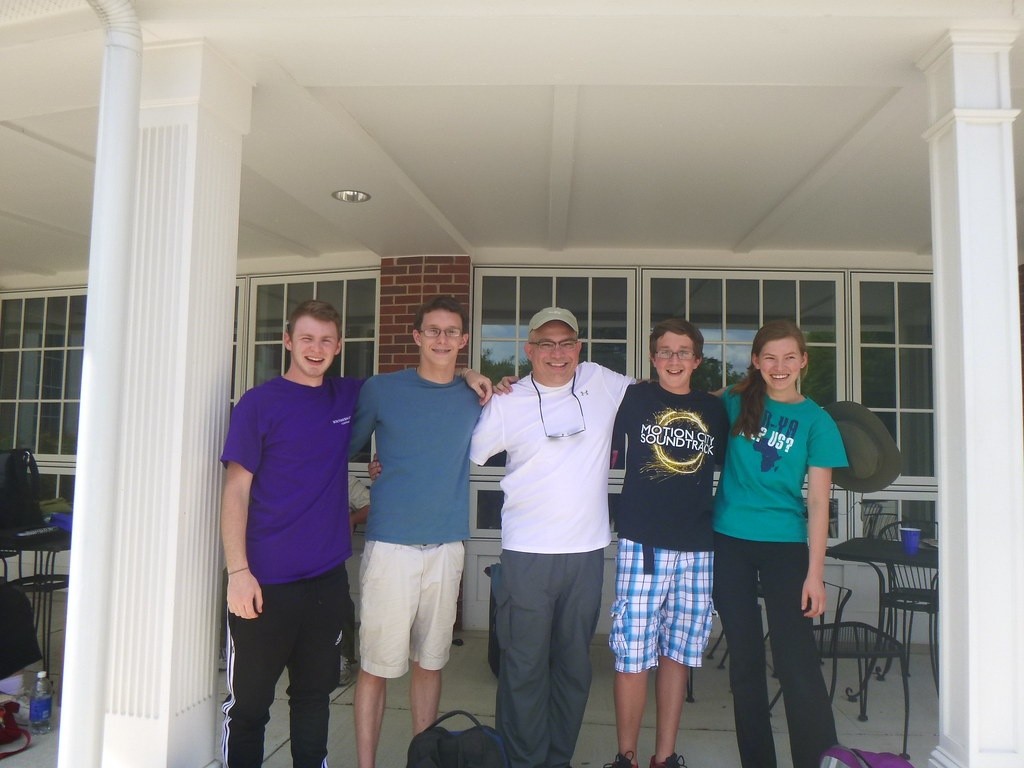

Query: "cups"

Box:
900,528,922,554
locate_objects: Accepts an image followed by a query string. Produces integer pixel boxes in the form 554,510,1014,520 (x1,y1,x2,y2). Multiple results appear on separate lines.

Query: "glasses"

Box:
655,349,696,361
528,338,580,353
419,327,464,338
540,396,586,438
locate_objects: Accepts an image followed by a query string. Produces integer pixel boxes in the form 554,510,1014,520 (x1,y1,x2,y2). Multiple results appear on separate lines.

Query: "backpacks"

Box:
0,447,43,530
406,711,510,768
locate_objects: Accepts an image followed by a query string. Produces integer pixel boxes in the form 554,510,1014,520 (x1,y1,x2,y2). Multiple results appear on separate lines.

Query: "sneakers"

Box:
338,656,351,685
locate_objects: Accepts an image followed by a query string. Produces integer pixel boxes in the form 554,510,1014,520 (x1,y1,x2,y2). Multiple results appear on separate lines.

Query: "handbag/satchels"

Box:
819,744,915,768
0,699,31,760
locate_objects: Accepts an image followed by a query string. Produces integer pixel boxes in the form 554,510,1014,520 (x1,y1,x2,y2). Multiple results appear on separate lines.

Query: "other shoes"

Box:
0,674,24,695
650,753,687,768
603,751,638,768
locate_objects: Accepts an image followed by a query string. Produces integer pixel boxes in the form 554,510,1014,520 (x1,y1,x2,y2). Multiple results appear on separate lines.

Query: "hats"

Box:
528,306,579,335
822,401,903,493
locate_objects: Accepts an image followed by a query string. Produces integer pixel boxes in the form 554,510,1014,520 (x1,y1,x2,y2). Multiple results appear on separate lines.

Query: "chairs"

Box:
686,508,938,758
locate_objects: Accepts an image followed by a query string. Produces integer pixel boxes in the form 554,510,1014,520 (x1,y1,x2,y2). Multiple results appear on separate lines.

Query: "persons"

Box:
217,475,370,685
711,321,850,768
494,319,729,768
219,300,492,767
0,576,43,726
368,307,727,768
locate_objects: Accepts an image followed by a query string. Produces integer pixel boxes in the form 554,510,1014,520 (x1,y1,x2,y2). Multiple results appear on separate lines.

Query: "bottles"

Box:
30,671,52,735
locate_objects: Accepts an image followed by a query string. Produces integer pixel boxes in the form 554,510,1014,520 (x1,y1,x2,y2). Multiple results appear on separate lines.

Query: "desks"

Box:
823,536,939,703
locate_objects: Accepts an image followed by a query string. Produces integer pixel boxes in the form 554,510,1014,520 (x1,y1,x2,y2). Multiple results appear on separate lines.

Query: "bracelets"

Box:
461,368,472,377
226,567,250,575
